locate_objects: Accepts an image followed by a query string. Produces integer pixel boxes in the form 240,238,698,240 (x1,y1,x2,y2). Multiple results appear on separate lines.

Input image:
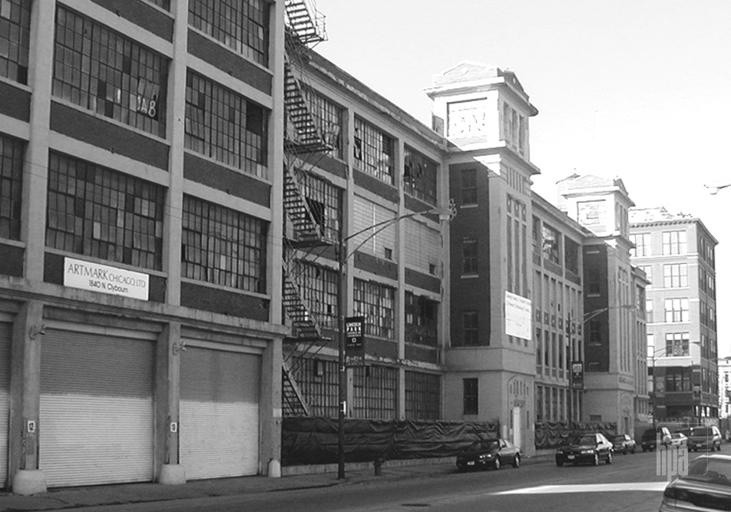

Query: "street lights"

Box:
569,303,637,427
337,202,453,486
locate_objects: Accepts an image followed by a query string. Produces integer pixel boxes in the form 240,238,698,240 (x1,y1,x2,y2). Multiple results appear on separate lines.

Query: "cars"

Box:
641,427,671,453
657,454,731,512
610,434,638,454
687,426,722,453
554,432,614,466
671,433,687,452
456,437,524,475
